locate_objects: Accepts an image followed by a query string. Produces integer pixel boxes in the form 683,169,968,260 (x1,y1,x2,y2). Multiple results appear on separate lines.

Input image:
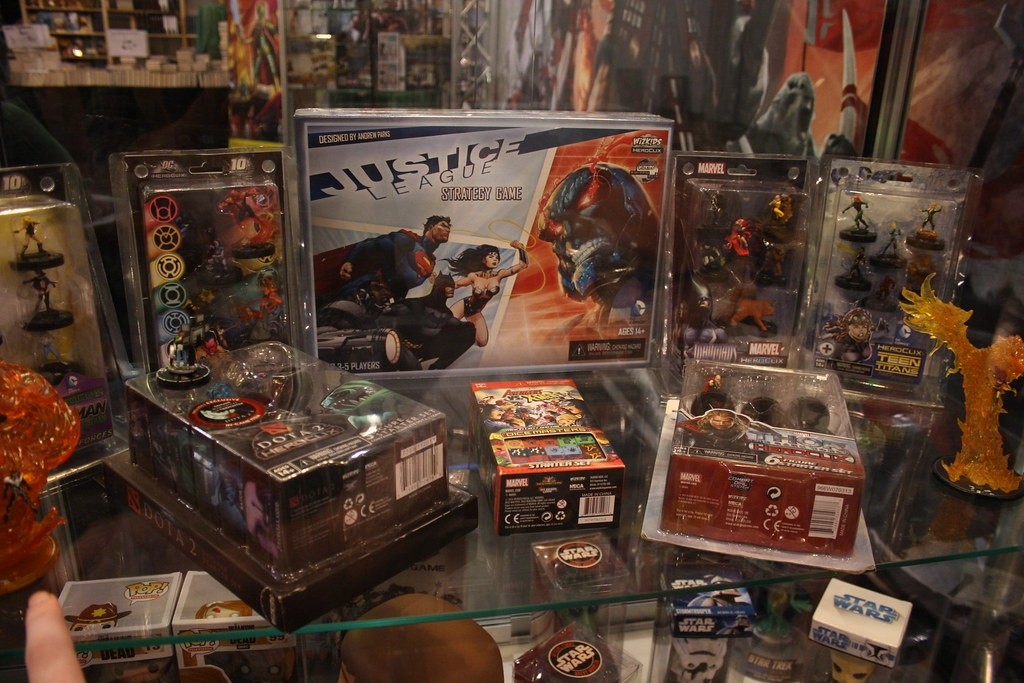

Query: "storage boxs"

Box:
123,341,448,585
807,578,913,669
468,379,627,537
637,356,877,577
514,621,644,683
289,106,679,381
528,531,631,628
55,570,179,683
658,572,757,639
102,448,480,632
170,570,304,683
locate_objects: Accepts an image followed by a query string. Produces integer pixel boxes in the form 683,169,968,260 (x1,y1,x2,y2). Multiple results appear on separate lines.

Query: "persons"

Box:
14,216,50,259
34,330,68,367
875,276,896,301
844,247,863,282
880,222,903,259
917,202,943,232
842,195,870,232
23,269,58,316
